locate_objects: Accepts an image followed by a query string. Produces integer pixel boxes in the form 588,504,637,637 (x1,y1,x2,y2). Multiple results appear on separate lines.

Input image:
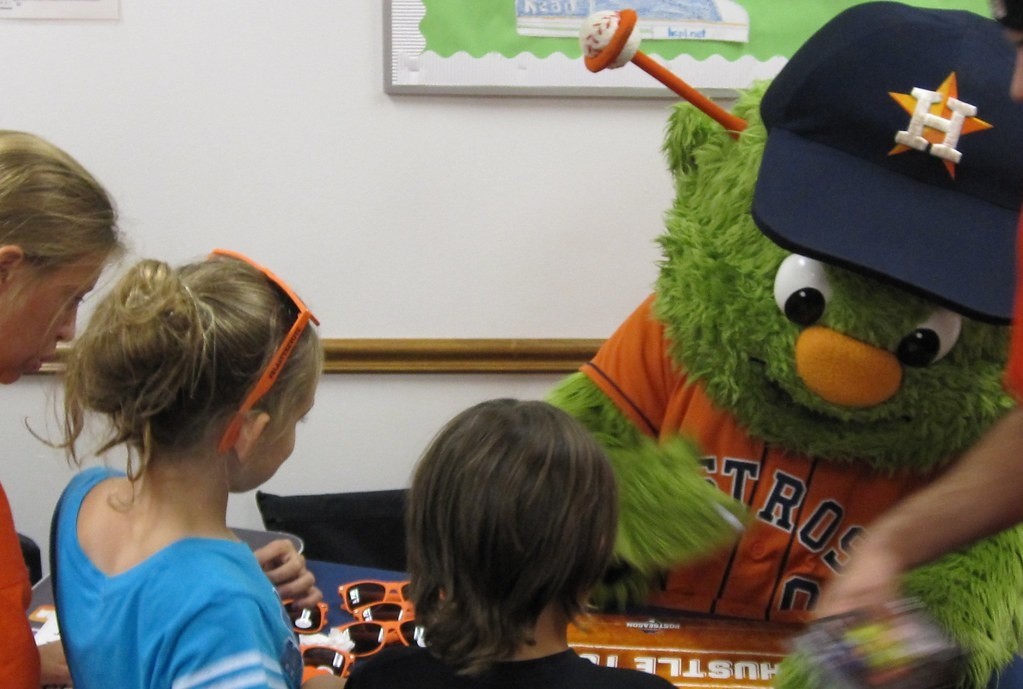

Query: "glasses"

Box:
206,249,321,452
280,579,421,682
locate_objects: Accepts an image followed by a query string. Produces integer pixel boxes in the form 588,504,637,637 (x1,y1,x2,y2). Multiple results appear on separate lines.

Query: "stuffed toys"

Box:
545,0,1023,689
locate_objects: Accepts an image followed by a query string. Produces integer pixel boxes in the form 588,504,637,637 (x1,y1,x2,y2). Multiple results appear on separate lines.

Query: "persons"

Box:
0,130,118,689
344,398,677,689
813,0,1023,611
25,248,349,689
